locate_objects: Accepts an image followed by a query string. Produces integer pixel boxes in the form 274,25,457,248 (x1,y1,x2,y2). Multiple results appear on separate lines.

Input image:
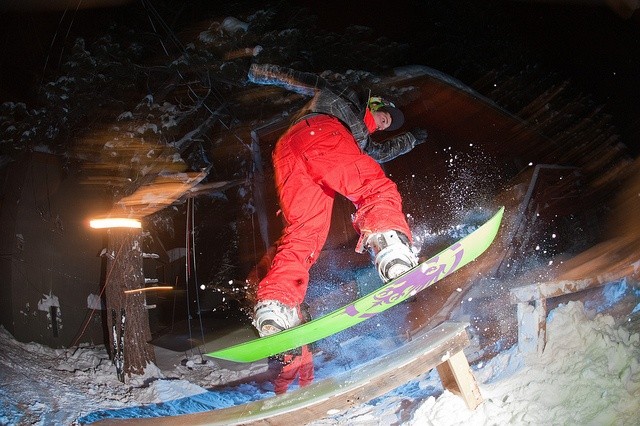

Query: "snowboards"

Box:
201,205,505,363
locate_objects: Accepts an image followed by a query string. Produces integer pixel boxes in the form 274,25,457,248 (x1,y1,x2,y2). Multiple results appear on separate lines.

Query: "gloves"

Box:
414,125,427,145
218,61,248,85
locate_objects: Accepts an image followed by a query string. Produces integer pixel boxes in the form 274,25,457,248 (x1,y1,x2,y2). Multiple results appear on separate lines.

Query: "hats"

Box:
370,97,404,132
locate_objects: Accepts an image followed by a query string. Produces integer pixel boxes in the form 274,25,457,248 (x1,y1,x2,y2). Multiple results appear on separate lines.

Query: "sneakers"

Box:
378,247,417,279
254,299,291,333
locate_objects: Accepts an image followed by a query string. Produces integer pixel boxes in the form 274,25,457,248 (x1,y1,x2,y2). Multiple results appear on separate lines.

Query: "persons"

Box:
267,303,315,396
219,61,429,340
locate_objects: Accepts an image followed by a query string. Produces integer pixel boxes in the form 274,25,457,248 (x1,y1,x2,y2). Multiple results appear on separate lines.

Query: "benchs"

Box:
509,252,637,356
83,319,485,426
